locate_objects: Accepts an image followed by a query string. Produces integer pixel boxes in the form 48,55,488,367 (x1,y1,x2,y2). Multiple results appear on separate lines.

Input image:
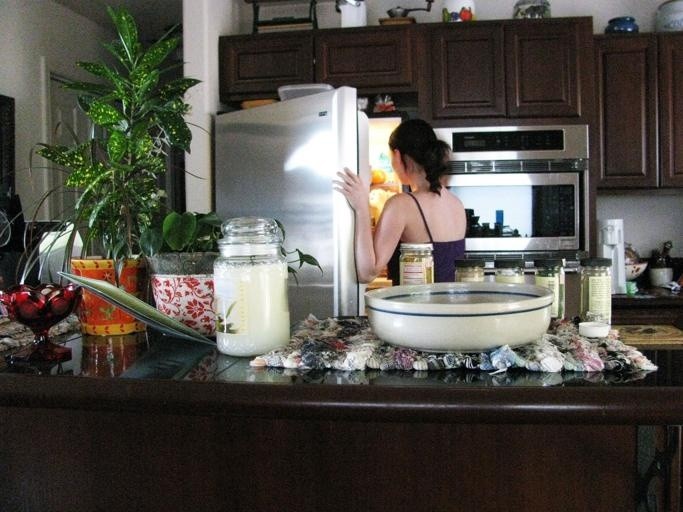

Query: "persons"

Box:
331,118,466,286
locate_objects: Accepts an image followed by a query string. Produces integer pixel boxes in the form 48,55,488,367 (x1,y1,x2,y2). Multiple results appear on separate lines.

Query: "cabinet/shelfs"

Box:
419,15,594,129
594,30,683,189
218,22,418,119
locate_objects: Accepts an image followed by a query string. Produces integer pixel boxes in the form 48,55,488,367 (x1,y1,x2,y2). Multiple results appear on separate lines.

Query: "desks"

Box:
612,287,683,325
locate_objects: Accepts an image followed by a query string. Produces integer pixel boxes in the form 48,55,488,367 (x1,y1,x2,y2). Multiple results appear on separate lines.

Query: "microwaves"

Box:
439,173,579,253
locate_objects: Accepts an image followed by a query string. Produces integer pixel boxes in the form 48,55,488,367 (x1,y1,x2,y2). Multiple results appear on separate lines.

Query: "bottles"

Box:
212,215,292,357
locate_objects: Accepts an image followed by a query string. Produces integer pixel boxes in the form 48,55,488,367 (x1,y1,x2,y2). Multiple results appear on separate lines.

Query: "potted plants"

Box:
139,209,324,340
0,4,209,336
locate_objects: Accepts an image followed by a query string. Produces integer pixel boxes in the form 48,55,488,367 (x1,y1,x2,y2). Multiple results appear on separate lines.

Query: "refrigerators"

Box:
213,86,408,319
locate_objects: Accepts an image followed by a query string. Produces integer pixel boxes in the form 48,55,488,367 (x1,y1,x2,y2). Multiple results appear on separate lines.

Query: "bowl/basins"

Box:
578,321,611,337
624,262,648,281
365,281,555,352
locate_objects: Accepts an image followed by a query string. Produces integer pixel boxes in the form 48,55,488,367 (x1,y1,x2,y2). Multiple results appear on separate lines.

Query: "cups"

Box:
650,268,673,287
